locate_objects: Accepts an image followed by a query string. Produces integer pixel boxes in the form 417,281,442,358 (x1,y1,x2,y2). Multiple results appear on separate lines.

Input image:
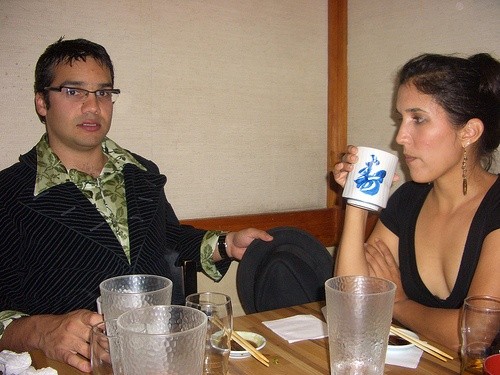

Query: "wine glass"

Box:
91,318,120,375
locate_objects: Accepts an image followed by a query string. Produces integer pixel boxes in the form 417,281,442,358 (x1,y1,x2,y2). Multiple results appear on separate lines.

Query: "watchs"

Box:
217,230,236,262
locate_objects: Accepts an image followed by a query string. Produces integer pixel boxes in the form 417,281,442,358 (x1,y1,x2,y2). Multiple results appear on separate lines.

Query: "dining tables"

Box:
28,300,462,375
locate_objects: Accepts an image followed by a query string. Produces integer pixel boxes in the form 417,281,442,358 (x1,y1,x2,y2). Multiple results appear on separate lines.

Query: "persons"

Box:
0,38,273,373
333,52,500,358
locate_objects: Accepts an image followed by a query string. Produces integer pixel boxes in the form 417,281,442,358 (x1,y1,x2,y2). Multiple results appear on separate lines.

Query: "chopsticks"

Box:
390,326,454,362
209,314,270,367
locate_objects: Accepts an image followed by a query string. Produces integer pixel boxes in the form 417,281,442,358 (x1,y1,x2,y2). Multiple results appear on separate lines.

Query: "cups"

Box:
117,304,208,375
325,275,397,375
185,291,233,375
342,147,399,211
99,275,173,375
96,296,142,335
460,295,500,375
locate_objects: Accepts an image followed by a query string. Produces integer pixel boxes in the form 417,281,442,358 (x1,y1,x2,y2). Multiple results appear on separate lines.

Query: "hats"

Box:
236,226,335,315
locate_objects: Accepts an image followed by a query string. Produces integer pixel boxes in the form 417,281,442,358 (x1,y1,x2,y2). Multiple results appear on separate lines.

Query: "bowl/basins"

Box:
483,354,500,375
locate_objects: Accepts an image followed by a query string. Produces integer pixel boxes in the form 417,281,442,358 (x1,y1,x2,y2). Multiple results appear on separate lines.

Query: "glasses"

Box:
44,86,120,104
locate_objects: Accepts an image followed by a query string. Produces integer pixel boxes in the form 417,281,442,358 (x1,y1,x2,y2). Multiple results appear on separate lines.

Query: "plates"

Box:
388,328,419,350
210,330,267,359
